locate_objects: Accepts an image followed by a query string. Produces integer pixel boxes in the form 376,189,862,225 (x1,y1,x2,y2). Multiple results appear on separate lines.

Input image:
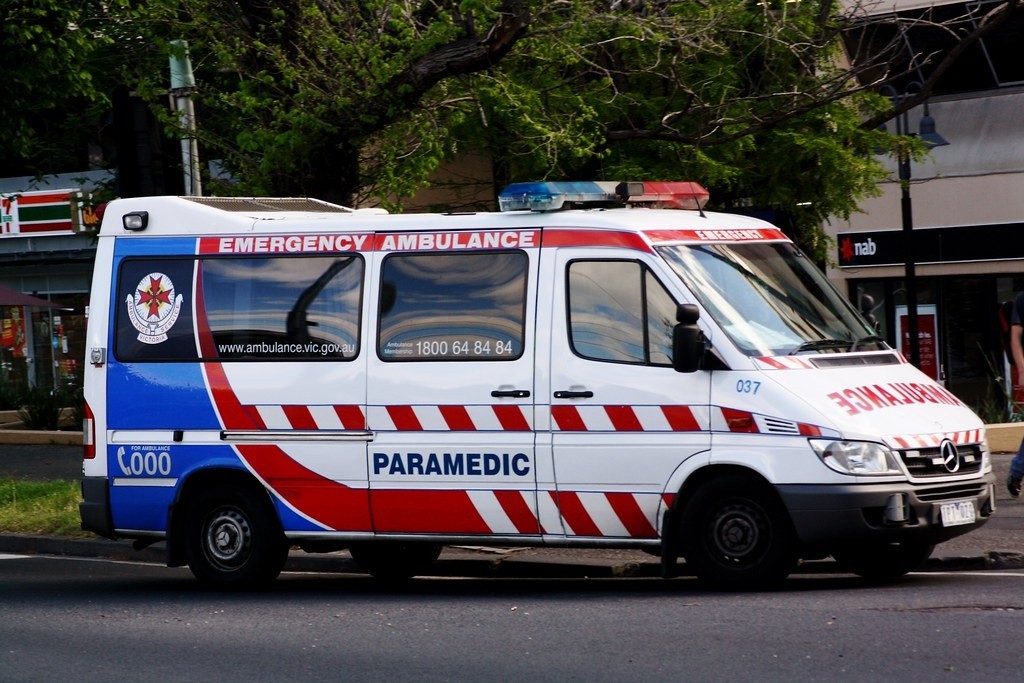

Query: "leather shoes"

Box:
1007,475,1021,498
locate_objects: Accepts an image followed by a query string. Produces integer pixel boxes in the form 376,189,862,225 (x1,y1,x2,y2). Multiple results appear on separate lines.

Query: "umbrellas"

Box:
0,283,59,307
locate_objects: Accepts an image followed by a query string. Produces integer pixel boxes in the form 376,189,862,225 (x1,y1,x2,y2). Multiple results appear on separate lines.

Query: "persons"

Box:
1005,289,1023,497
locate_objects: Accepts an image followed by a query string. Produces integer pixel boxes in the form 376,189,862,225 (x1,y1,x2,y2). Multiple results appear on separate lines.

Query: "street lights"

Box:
880,81,950,370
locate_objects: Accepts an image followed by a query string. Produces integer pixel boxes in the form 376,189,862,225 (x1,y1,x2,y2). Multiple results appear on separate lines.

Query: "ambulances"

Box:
81,179,995,600
